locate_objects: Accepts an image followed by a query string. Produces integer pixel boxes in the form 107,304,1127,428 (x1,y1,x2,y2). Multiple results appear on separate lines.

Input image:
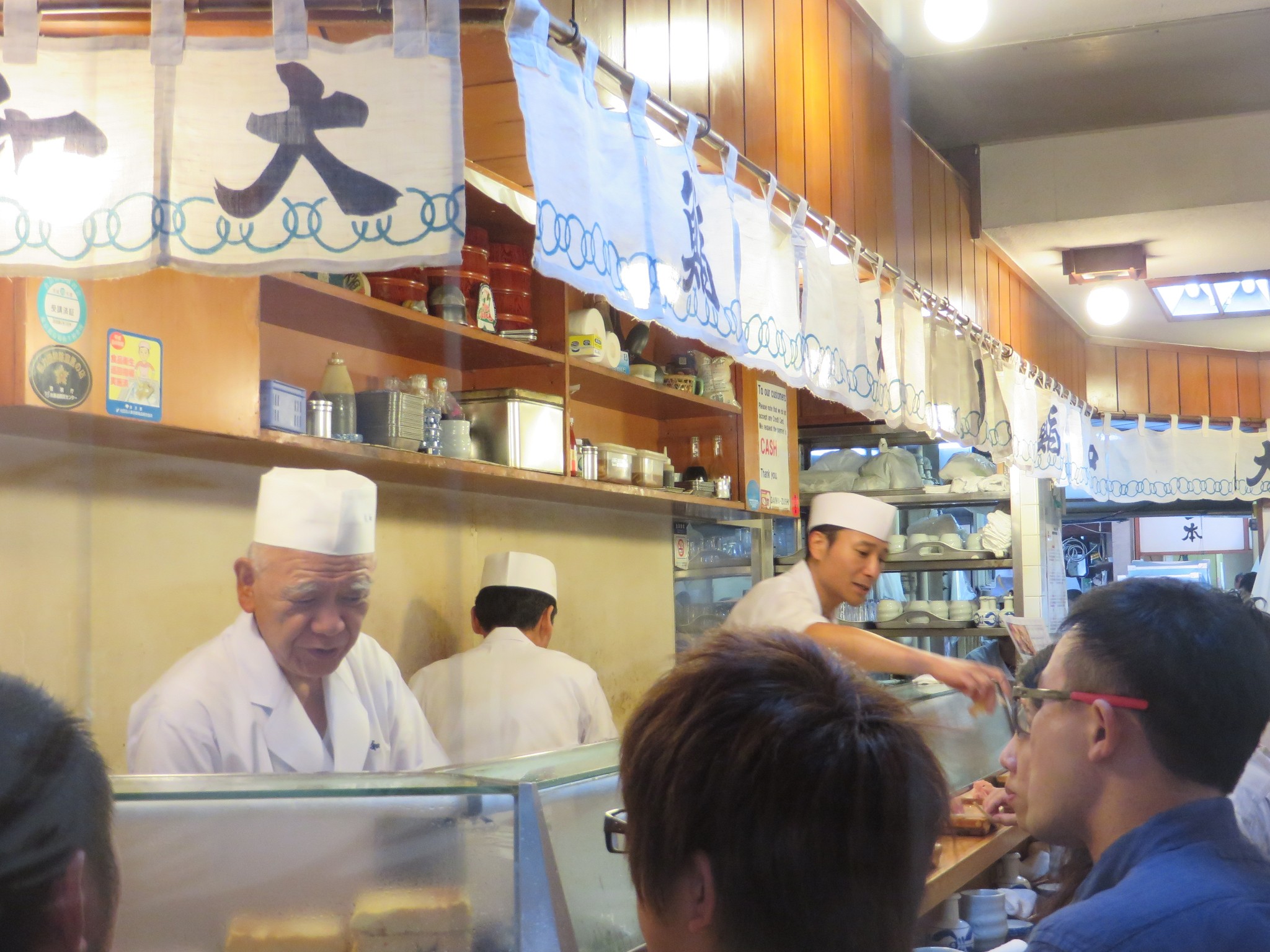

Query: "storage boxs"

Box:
451,387,568,477
592,443,637,486
632,449,669,490
260,379,307,434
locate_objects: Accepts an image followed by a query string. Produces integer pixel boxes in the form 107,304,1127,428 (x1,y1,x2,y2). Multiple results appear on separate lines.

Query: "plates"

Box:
353,388,426,452
1038,882,1063,895
674,478,714,499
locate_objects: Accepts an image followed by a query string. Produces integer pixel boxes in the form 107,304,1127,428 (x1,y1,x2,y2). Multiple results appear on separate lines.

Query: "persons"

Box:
410,547,620,867
1066,589,1084,617
718,491,1014,716
615,623,952,952
982,740,1270,859
964,636,1017,686
2,670,125,951
1001,643,1096,926
1238,571,1259,608
1022,577,1269,950
1234,575,1243,588
122,459,469,888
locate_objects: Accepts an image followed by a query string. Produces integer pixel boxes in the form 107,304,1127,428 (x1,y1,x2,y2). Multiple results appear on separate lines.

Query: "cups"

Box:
961,888,1009,951
686,595,740,625
831,524,1026,630
684,528,782,571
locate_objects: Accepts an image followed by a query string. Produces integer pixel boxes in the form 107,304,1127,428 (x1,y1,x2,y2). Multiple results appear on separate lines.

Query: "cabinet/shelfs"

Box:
796,425,1013,638
0,155,798,523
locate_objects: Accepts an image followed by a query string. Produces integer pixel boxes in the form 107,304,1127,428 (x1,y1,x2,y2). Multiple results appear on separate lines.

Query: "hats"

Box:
253,467,378,557
478,551,557,601
807,492,900,543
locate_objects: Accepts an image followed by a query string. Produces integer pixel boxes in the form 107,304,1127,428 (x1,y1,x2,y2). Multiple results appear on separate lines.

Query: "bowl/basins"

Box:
410,406,477,460
1005,917,1034,943
1004,886,1039,920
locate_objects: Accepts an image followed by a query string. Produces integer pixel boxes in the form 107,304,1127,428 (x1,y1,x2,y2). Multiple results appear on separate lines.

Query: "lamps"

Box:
1061,245,1147,325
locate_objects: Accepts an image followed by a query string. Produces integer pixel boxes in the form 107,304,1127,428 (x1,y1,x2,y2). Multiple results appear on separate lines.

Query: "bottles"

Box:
684,436,708,483
318,353,357,441
709,435,732,501
928,891,974,952
662,445,675,487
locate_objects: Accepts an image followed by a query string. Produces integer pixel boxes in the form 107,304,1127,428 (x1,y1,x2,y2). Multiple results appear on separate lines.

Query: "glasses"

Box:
1010,683,1151,739
604,806,631,858
